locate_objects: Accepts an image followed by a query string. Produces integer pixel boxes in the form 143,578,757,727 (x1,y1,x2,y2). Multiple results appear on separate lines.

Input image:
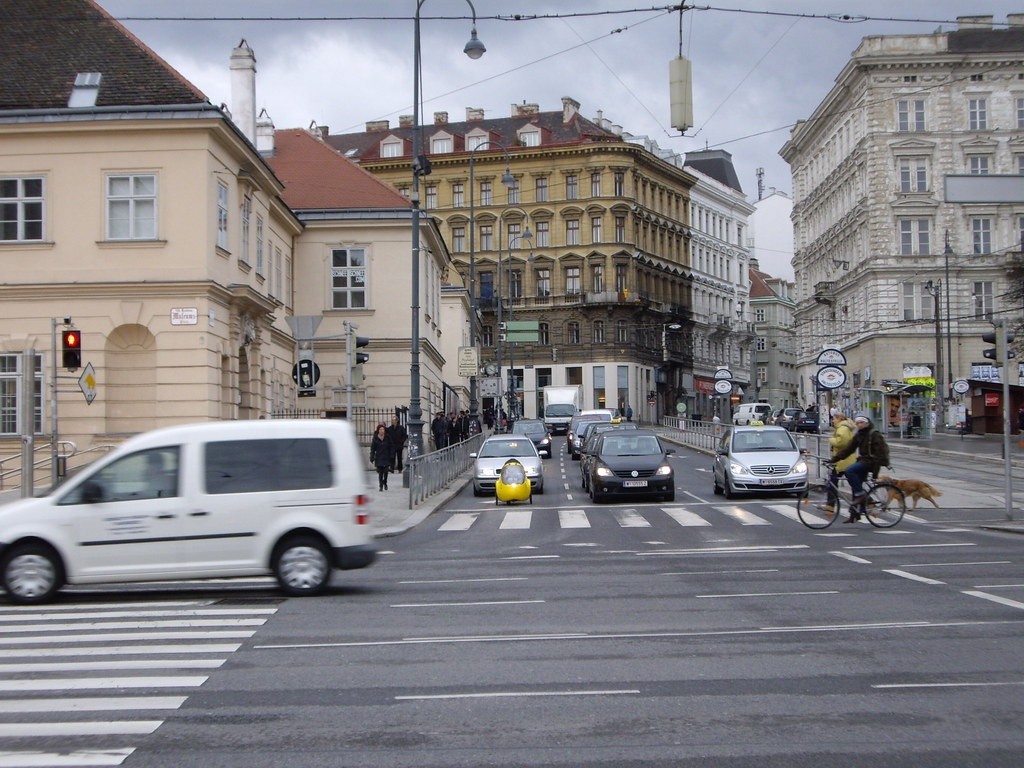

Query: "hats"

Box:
854,413,869,424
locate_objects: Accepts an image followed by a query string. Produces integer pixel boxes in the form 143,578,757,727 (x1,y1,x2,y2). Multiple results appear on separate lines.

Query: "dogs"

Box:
873,475,944,509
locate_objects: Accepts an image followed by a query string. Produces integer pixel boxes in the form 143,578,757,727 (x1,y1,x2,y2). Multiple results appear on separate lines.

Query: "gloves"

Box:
830,456,838,463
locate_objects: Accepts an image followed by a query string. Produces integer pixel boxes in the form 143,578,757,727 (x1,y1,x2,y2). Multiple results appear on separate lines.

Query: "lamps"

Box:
670,3,694,135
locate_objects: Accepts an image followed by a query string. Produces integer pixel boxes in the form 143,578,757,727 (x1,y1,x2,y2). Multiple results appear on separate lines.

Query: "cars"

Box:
511,420,552,460
761,408,819,434
564,410,676,502
469,433,545,495
711,420,811,497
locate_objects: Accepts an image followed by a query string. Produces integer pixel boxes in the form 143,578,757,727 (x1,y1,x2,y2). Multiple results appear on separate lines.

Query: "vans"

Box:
0,418,379,604
732,403,772,426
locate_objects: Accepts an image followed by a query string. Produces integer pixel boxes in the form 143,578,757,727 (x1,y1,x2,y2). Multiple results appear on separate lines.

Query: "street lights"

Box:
404,0,485,454
468,141,517,430
507,237,535,422
943,228,957,399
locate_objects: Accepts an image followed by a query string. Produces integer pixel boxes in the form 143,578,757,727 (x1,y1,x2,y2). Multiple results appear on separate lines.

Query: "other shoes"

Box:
843,513,860,523
850,490,867,505
821,504,835,512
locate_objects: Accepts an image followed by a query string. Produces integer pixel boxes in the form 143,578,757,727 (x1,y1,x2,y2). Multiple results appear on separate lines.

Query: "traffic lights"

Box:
350,333,369,367
61,330,81,368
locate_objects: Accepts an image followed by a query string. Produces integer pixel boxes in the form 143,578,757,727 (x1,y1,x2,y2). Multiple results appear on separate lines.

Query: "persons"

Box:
820,412,858,513
136,453,175,499
626,405,633,423
432,408,507,450
370,425,395,491
805,403,839,419
825,414,892,524
387,416,408,474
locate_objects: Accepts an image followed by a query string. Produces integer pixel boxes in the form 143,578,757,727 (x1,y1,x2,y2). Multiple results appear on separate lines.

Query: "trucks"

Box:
543,384,584,434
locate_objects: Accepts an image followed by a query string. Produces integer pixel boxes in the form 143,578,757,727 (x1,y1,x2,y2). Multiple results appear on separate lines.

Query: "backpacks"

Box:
868,429,889,467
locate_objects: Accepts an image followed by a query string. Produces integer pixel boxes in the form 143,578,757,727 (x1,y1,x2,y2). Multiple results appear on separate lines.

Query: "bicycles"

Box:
796,455,906,529
